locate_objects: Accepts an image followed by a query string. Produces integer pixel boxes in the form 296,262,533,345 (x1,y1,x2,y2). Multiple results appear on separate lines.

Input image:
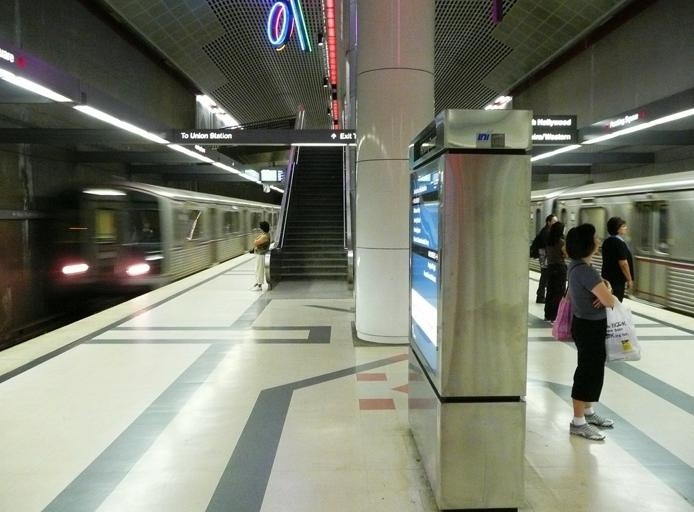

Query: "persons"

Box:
248,222,270,290
544,222,568,323
536,215,558,303
566,223,618,441
602,217,634,303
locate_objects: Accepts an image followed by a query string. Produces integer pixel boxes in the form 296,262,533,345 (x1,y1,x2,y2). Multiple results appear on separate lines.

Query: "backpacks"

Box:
530,237,539,259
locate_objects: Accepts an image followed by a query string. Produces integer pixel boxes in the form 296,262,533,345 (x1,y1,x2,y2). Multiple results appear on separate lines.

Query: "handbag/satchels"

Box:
605,295,642,361
552,297,573,342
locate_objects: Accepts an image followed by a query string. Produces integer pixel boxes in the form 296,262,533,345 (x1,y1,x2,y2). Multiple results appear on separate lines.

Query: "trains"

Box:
529,171,694,314
61,181,281,292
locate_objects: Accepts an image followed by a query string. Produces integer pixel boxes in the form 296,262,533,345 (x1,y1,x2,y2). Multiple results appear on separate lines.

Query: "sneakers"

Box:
570,413,614,440
248,283,262,291
